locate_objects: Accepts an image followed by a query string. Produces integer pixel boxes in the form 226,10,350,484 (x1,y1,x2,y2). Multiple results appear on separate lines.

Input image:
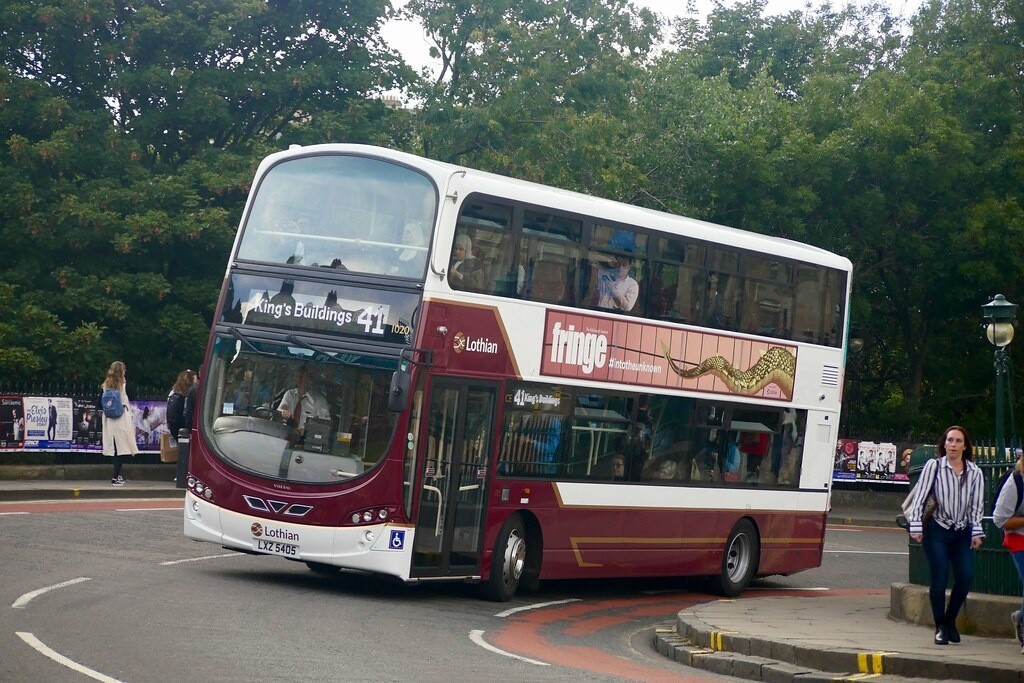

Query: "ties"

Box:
291,396,307,430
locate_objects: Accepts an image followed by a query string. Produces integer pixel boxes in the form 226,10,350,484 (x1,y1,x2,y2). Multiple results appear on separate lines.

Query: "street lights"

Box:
982,294,1019,495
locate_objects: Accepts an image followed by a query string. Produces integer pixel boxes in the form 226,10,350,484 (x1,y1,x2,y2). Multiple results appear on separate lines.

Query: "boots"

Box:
934,614,949,645
945,604,961,642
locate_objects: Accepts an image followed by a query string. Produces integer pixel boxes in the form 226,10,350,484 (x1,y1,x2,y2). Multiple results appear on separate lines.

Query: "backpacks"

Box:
102,388,124,419
167,393,184,425
992,469,1023,519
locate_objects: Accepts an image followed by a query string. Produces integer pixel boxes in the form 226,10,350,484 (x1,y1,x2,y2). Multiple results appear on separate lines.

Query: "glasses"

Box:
610,464,622,468
455,247,465,251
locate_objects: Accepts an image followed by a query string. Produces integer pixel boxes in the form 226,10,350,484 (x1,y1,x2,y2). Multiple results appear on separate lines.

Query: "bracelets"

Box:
460,275,463,280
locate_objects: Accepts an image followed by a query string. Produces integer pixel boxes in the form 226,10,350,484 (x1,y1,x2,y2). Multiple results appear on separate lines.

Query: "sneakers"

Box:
111,479,122,486
1010,608,1023,652
117,476,124,482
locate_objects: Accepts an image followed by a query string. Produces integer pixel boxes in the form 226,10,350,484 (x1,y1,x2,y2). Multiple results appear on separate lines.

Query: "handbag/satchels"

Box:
157,430,177,464
906,493,939,532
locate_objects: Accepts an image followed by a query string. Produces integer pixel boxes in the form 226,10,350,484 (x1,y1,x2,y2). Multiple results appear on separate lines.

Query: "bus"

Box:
182,143,853,601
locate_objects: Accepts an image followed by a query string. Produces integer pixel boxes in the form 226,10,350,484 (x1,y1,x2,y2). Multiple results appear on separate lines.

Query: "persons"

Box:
835,441,851,471
392,223,426,276
167,368,269,488
138,406,155,447
99,361,138,486
47,399,57,440
877,451,895,472
610,455,624,477
597,254,641,311
516,413,562,461
859,450,874,471
710,429,741,482
902,425,987,645
447,235,485,288
992,449,1024,654
269,366,330,435
7,410,24,440
